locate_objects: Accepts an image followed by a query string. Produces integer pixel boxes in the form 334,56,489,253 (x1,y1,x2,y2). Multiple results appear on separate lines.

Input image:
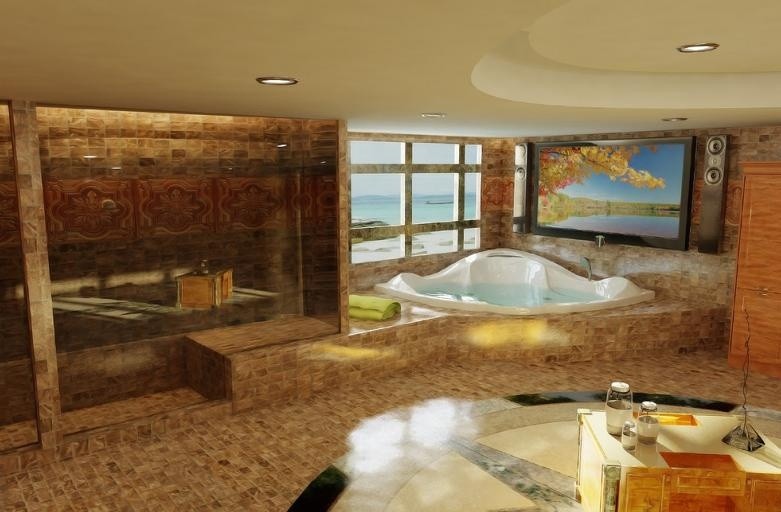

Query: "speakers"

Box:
697,133,733,255
512,142,536,234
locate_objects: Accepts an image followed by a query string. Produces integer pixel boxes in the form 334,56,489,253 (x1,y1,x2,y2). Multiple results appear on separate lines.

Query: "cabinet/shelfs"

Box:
727,160,781,378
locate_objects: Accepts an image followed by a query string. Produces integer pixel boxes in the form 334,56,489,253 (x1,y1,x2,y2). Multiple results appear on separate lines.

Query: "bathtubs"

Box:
374,247,656,316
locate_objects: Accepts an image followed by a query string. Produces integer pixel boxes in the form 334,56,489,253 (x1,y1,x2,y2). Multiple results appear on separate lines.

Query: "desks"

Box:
176,266,236,310
575,407,781,512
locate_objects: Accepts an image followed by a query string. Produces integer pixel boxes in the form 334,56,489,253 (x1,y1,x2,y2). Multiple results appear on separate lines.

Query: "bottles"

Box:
635,400,660,447
605,381,633,437
622,420,637,452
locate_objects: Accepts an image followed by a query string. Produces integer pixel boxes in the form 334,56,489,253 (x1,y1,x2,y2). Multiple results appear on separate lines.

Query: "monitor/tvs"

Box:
530,136,695,252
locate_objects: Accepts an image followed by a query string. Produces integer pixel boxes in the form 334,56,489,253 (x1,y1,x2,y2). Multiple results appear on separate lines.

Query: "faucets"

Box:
582,256,592,280
